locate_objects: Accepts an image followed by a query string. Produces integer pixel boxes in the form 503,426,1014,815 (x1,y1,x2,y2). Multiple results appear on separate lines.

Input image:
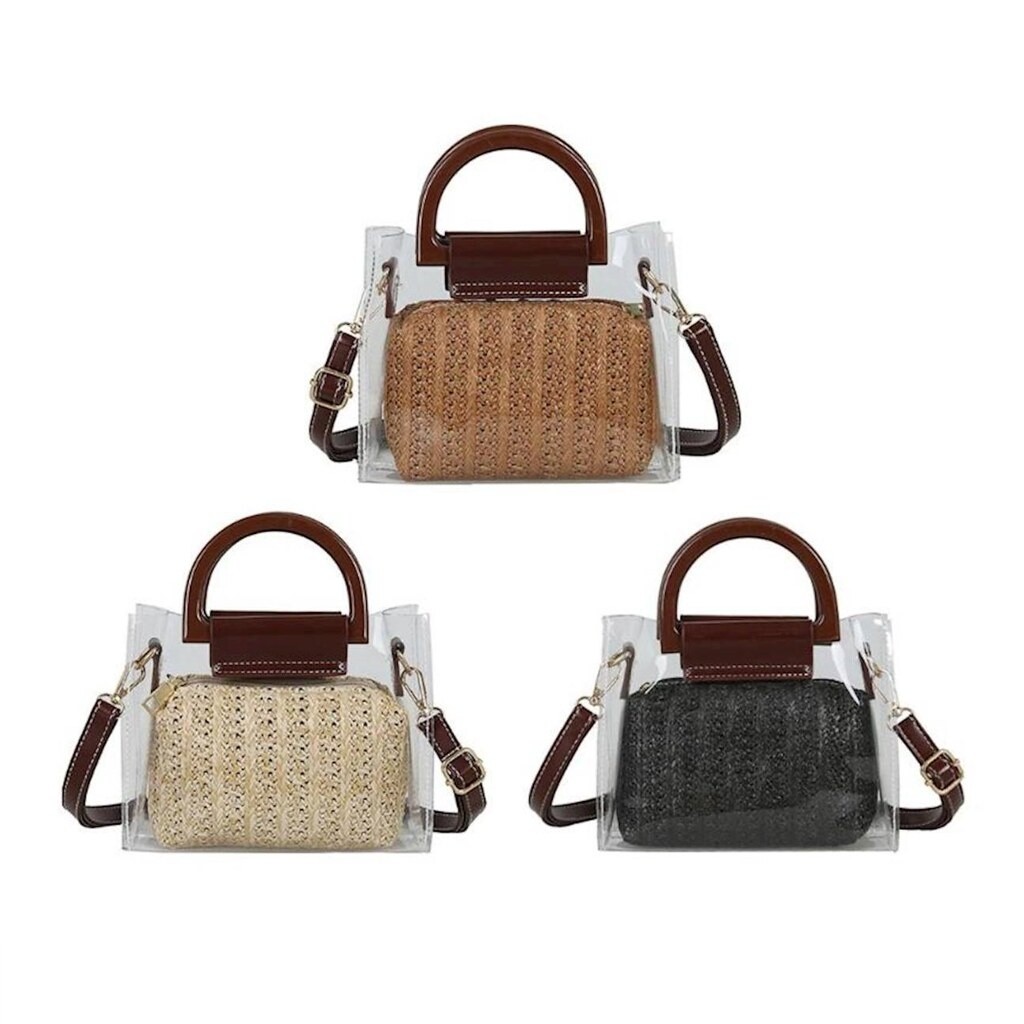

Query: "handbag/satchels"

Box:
60,510,487,854
308,125,743,484
531,516,966,853
